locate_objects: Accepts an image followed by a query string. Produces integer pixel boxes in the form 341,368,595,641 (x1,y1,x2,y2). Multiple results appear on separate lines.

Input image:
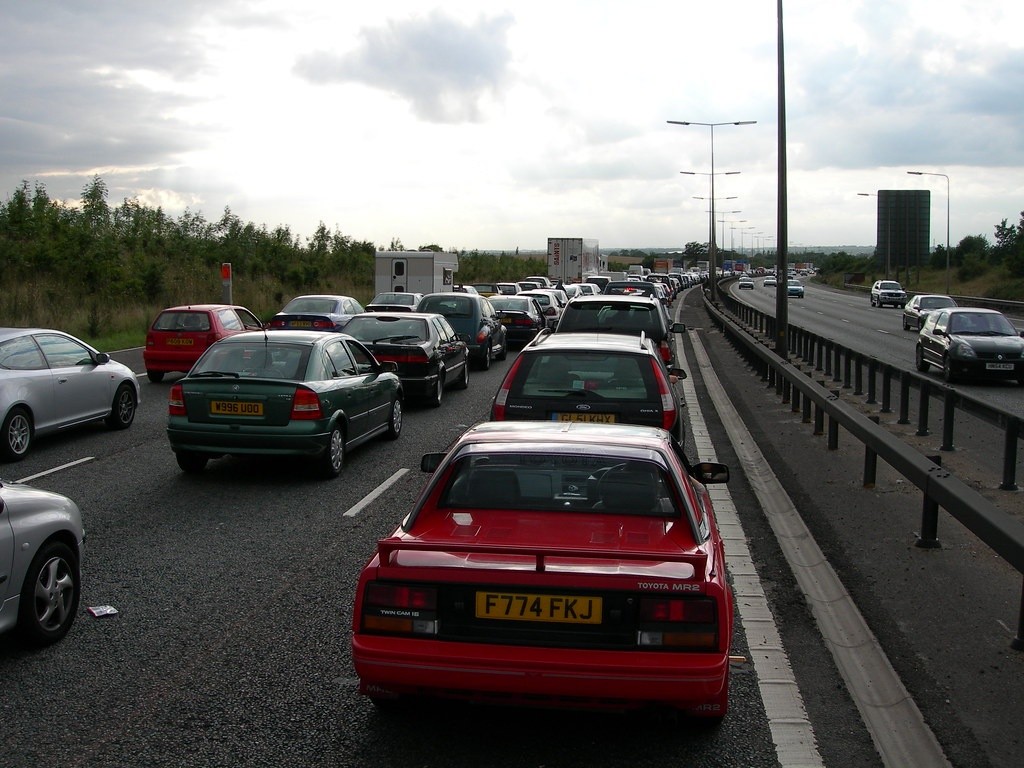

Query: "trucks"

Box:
547,237,605,284
375,252,459,295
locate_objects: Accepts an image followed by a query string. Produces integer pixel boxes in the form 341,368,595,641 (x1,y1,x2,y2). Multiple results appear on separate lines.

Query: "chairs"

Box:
470,463,543,509
594,463,661,515
183,315,201,327
633,308,650,322
537,362,570,391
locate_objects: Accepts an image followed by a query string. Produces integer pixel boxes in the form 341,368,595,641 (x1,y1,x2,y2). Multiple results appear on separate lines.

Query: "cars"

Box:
915,307,1024,385
454,275,552,297
902,294,959,332
142,304,262,383
0,478,86,647
738,274,755,289
487,276,613,352
763,276,777,287
788,269,814,280
268,294,367,331
415,292,508,371
1,327,140,464
788,280,804,298
352,420,731,733
332,311,471,408
600,268,767,311
366,292,423,313
166,328,404,480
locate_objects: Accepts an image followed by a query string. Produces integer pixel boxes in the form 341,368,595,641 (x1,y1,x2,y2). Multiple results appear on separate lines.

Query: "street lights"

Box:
680,167,772,294
667,121,757,303
857,193,891,276
907,172,950,294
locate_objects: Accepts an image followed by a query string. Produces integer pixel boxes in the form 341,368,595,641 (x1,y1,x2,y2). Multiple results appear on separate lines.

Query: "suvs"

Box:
870,280,907,308
555,292,687,369
490,326,688,449
